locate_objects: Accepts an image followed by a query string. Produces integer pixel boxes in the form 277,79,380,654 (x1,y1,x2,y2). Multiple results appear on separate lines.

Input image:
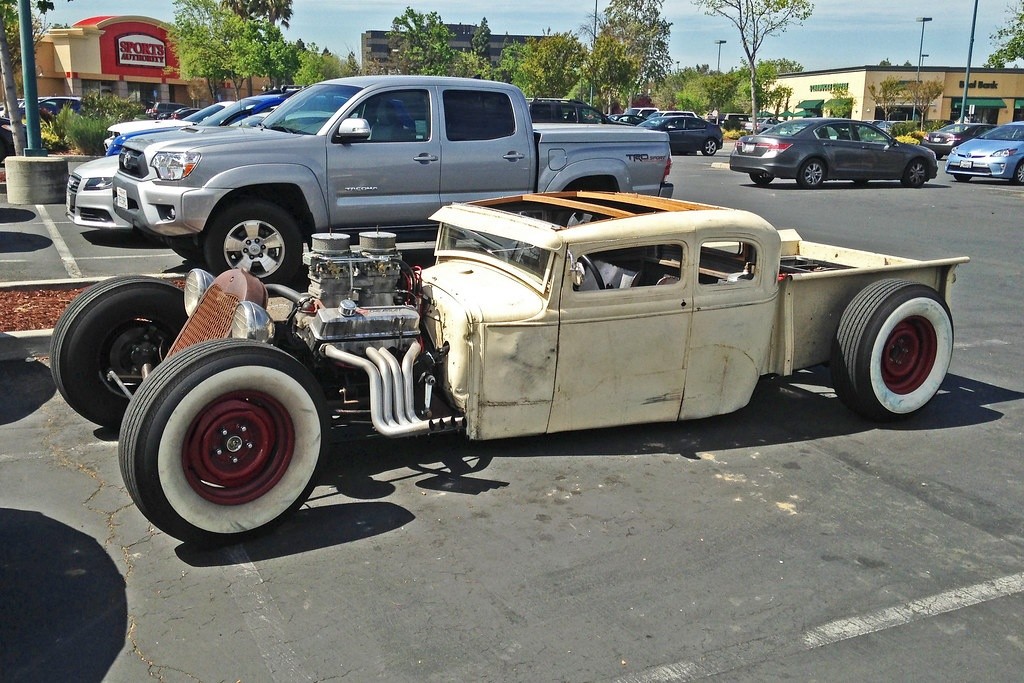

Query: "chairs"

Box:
838,131,850,141
818,128,829,139
368,99,416,140
573,217,656,292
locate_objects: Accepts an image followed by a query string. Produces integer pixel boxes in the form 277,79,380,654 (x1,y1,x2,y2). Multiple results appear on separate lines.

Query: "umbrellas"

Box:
756,110,815,117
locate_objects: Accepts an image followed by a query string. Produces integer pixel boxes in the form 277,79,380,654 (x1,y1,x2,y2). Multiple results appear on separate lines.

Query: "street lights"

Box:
920,54,931,68
675,61,681,74
912,17,934,121
714,40,727,108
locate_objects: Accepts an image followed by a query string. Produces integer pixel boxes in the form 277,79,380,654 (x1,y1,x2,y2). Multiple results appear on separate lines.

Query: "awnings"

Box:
954,99,1007,108
876,99,935,107
795,100,824,109
822,99,851,108
1014,100,1024,109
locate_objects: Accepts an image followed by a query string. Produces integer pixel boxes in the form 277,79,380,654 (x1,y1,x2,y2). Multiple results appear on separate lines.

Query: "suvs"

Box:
524,98,634,127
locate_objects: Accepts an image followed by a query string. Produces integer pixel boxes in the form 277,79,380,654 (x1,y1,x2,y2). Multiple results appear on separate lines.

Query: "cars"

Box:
862,119,882,126
728,118,939,188
740,117,782,133
622,107,659,119
0,85,416,246
945,121,1024,186
635,115,723,156
877,120,906,135
605,114,647,127
644,110,699,121
923,123,997,159
47,188,973,550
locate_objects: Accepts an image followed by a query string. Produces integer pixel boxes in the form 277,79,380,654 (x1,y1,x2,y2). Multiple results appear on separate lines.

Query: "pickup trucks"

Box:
110,74,676,290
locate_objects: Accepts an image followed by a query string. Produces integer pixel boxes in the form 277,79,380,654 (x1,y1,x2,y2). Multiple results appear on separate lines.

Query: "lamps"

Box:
867,106,871,112
